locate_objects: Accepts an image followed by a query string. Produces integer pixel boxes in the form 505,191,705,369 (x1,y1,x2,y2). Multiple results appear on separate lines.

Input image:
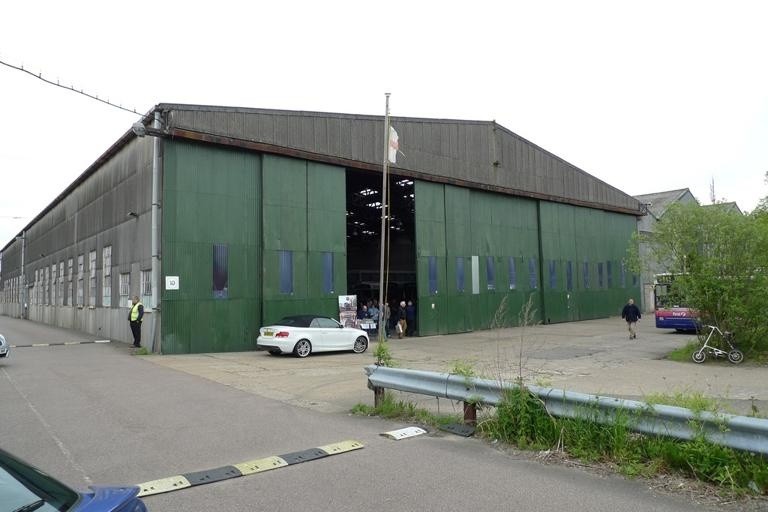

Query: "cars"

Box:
256,314,369,358
0,447,146,512
0,333,9,358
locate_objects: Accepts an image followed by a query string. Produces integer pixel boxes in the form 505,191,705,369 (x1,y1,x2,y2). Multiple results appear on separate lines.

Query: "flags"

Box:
388,125,398,163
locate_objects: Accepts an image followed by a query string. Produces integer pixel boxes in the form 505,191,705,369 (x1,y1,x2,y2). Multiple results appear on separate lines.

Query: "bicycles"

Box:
691,325,744,364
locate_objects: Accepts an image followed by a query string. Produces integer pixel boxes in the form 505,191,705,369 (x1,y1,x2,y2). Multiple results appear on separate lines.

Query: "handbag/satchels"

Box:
395,321,403,335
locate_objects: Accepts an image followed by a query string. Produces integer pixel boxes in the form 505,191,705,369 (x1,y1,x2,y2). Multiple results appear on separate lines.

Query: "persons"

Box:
397,301,407,339
343,297,353,311
127,296,144,347
622,298,642,340
405,301,417,337
356,299,392,338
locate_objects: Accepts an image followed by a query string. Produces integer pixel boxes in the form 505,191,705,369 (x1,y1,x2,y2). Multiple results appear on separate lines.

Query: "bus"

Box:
653,272,754,334
